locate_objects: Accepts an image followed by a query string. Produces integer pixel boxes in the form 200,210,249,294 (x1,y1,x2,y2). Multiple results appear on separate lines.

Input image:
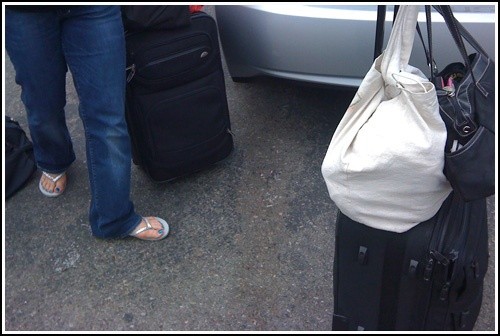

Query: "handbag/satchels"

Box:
321,4,453,232
5,115,36,200
415,4,494,202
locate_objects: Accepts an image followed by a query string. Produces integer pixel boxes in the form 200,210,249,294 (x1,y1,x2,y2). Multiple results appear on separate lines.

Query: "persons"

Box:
5,6,170,242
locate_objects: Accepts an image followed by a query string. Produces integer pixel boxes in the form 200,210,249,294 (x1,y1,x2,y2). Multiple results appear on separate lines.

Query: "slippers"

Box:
38,171,68,197
128,215,170,241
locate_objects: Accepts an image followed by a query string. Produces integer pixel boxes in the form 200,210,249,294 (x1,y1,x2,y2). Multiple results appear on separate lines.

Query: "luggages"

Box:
330,195,488,329
124,10,235,182
